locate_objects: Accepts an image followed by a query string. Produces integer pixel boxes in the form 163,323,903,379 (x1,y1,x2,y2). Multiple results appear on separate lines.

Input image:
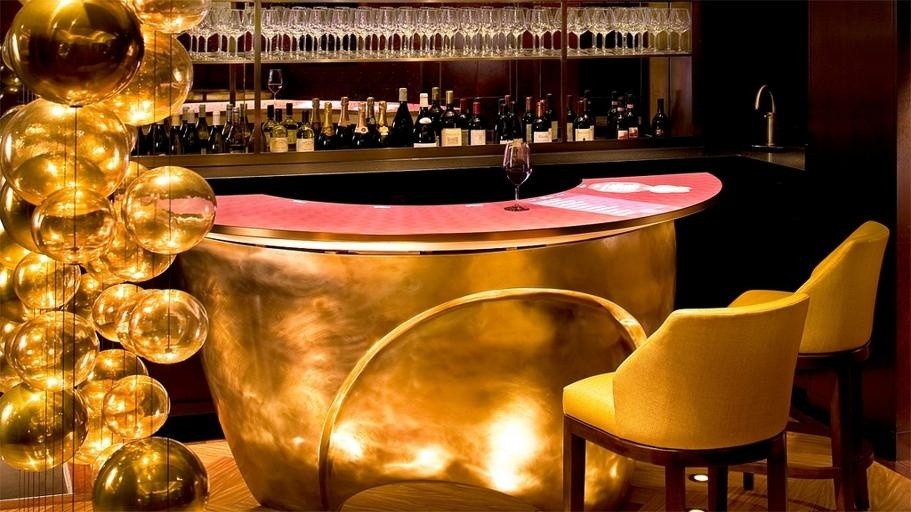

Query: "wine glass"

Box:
501,143,533,211
567,6,691,56
258,5,562,61
181,2,252,58
266,68,282,100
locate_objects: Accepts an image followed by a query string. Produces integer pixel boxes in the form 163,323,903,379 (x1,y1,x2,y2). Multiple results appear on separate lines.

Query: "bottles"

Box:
225,107,244,153
183,110,198,153
352,103,372,148
335,96,352,149
615,96,628,141
179,107,189,132
260,104,273,151
136,123,153,155
606,91,617,138
651,98,669,137
546,93,559,141
625,94,639,139
530,102,552,144
457,98,468,145
565,89,596,142
281,102,297,151
491,95,521,141
468,101,487,145
221,104,232,137
376,100,389,147
413,92,436,148
294,110,314,153
390,88,413,148
195,104,209,154
317,102,335,149
539,99,547,117
429,88,442,125
158,116,178,153
364,97,376,131
439,90,460,147
238,103,252,152
210,110,223,153
269,108,288,153
309,98,321,134
521,96,533,141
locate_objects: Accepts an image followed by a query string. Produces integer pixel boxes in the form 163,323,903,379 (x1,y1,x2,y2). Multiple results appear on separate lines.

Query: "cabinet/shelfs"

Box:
126,1,701,169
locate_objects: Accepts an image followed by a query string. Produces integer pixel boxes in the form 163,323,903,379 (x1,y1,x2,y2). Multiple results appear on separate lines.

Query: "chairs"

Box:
715,220,892,511
562,288,808,512
